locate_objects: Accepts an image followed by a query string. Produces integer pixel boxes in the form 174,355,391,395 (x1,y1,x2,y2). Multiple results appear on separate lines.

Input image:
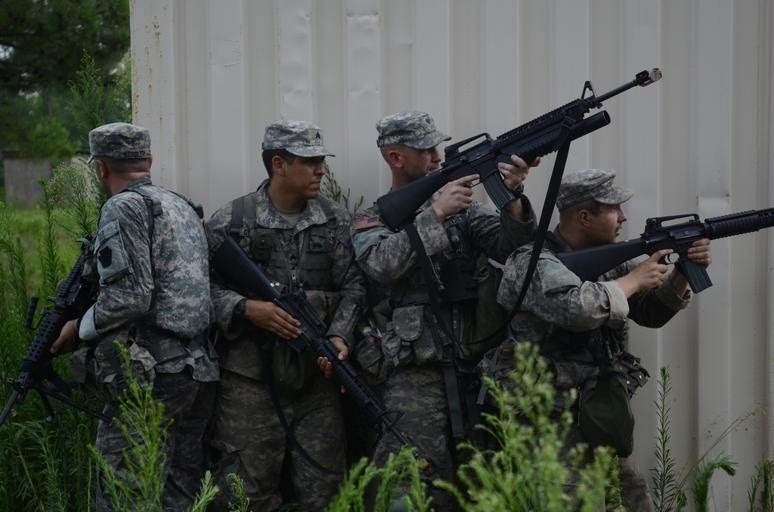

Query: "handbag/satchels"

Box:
575,370,635,457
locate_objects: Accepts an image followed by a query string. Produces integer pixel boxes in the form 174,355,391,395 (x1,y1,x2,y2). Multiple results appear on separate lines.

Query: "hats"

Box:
557,169,632,209
86,122,152,164
377,111,451,150
263,122,334,159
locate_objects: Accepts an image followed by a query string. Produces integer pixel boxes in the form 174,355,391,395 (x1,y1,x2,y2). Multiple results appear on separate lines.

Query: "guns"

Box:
0,233,110,429
376,69,662,233
210,236,420,458
555,207,773,293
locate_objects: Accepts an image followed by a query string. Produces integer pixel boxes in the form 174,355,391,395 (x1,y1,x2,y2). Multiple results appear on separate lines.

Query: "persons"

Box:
496,168,713,512
350,110,541,511
50,122,219,512
205,120,368,512
